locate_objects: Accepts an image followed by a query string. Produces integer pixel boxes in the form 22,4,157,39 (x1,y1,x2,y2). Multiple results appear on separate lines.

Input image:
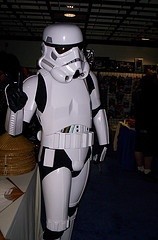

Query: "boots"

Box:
61,208,78,240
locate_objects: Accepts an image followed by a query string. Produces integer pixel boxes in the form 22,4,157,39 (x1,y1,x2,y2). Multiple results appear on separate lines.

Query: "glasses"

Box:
42,39,88,56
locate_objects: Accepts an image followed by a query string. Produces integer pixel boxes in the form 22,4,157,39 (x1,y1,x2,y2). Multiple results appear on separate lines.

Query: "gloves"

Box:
3,69,27,112
91,143,106,172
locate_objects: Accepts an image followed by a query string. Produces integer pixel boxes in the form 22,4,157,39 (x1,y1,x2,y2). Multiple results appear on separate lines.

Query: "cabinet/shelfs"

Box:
0,164,46,240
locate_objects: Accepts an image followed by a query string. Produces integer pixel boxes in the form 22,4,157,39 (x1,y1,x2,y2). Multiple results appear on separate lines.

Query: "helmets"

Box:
39,24,91,83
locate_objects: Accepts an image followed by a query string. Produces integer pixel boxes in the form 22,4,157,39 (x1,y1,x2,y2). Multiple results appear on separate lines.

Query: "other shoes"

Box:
136,165,151,175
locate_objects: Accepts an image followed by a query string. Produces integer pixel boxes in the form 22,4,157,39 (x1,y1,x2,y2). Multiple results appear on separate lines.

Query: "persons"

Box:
1,22,110,240
130,74,157,181
